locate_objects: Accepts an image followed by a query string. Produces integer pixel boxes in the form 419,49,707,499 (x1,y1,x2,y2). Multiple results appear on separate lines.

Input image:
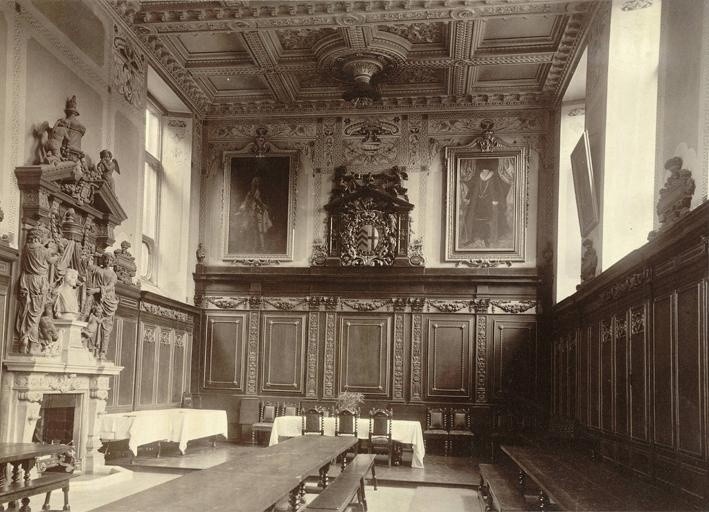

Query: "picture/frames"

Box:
444,130,530,267
570,129,600,236
220,137,298,267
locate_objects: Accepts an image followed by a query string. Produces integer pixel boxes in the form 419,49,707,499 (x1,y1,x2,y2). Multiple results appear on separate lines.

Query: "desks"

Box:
1,441,68,510
103,409,227,462
89,434,359,512
500,443,708,511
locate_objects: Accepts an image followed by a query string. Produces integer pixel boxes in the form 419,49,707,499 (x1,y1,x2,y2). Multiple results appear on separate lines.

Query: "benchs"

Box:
0,473,74,511
299,455,375,512
476,462,529,510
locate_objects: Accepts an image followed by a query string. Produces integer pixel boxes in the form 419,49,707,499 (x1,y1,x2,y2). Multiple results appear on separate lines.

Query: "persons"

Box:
463,160,509,250
13,99,124,369
229,174,275,254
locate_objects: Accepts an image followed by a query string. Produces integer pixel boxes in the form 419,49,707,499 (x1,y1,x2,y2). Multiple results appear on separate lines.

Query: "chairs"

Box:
251,401,518,466
